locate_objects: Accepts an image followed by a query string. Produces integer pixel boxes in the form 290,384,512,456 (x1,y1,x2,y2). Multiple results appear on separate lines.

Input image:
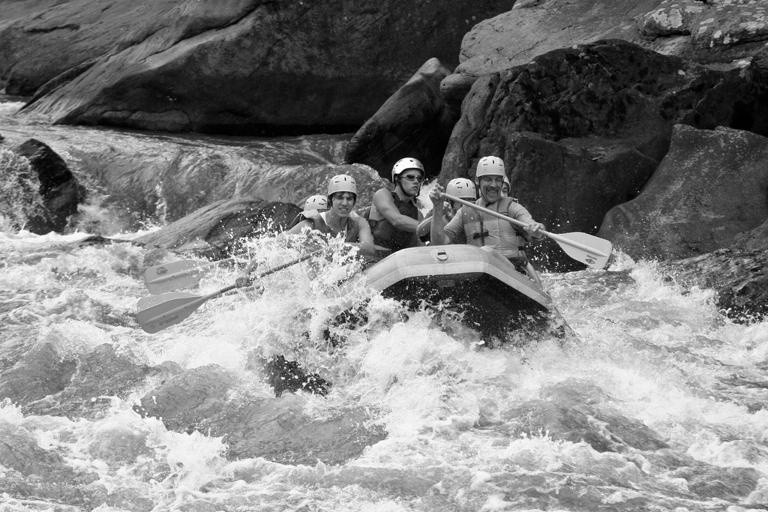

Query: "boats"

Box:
359,243,551,339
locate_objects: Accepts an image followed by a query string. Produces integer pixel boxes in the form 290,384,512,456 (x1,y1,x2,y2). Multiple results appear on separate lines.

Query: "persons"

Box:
304,193,334,214
416,176,476,245
499,173,512,197
429,154,546,272
369,155,433,262
280,173,376,270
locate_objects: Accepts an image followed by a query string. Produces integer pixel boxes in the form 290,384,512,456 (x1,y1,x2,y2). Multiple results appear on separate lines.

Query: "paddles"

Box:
134,248,322,334
439,191,614,270
145,254,247,295
137,287,256,315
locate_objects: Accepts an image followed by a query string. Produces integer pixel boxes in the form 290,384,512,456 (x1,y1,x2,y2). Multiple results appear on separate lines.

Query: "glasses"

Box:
399,175,423,183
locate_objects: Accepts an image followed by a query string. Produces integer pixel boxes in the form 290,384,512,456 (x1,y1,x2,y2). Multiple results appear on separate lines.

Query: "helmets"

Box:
476,156,511,192
392,157,425,182
300,195,329,219
446,178,476,200
327,174,357,205
